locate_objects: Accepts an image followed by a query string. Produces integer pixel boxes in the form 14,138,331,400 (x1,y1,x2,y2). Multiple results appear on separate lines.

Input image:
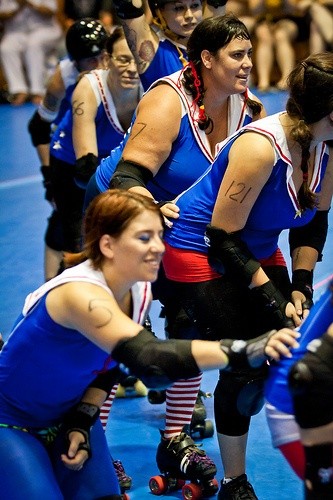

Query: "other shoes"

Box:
218,473,259,500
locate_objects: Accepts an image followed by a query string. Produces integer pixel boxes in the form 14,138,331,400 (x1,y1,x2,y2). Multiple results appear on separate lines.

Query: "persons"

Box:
153,51,332,499
279,270,333,500
115,0,232,114
1,184,303,500
0,0,333,190
43,23,157,291
73,11,277,438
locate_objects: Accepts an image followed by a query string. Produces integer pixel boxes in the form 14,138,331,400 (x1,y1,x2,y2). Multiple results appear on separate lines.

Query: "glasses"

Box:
108,54,136,66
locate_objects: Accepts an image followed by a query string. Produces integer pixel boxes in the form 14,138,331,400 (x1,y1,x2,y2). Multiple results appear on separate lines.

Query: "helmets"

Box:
65,17,110,61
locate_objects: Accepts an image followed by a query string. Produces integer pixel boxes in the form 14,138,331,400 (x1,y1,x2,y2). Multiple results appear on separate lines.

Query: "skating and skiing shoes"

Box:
147,389,214,440
149,427,218,500
113,461,132,500
